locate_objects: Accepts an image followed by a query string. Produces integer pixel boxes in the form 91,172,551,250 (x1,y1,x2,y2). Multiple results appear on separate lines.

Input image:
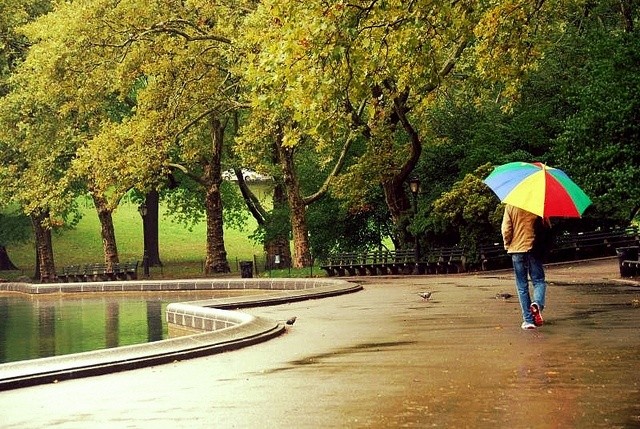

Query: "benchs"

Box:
319,244,468,276
58,260,140,282
477,226,640,270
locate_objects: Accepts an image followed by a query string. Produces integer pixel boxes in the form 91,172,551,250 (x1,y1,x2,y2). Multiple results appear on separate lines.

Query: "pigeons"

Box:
285,316,298,327
419,290,432,300
497,293,512,301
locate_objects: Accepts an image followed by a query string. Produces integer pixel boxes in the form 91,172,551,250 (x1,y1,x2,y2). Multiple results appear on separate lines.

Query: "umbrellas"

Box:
483,161,594,221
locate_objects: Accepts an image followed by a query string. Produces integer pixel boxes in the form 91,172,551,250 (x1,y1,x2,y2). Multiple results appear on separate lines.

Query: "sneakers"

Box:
521,320,536,330
530,301,544,325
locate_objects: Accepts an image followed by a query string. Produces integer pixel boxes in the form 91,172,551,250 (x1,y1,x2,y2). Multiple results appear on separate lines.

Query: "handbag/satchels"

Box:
532,215,557,251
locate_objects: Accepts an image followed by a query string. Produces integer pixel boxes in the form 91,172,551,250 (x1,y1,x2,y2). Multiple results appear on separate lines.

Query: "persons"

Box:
500,204,552,330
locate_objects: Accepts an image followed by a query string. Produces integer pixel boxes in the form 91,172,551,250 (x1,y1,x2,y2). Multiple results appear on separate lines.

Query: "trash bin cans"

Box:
239,261,253,277
